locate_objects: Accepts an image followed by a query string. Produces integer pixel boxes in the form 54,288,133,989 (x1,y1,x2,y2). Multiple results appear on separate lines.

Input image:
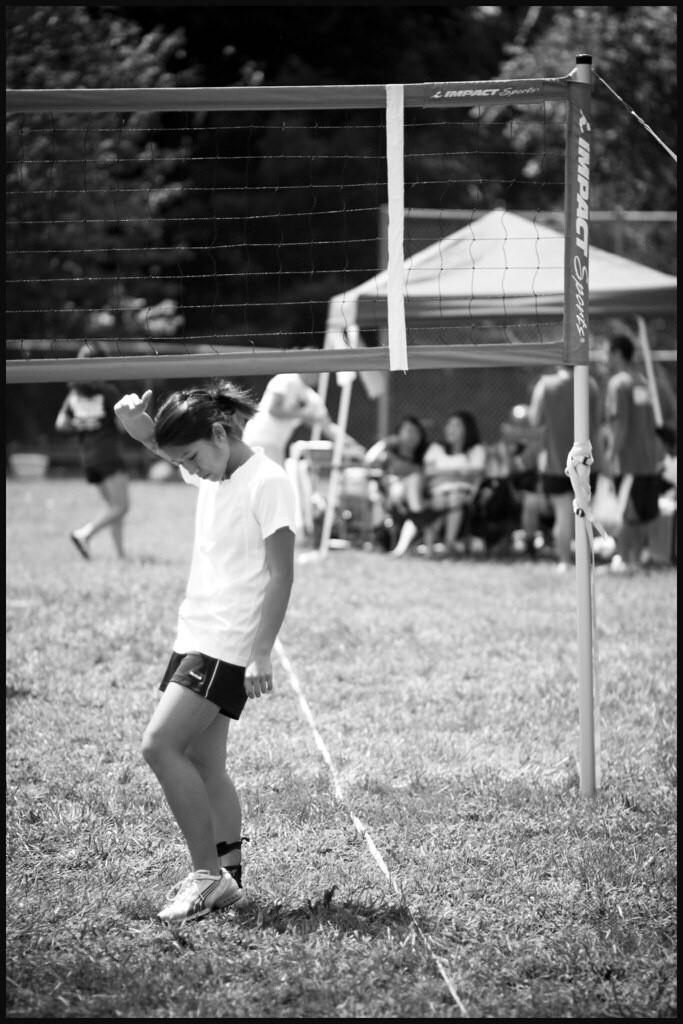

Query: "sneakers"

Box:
227,887,254,907
157,867,244,923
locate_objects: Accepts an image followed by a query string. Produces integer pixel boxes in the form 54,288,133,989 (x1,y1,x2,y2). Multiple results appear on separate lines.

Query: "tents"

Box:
307,207,683,554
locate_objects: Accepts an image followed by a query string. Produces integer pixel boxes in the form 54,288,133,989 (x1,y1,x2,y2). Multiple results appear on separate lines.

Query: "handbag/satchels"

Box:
592,469,624,538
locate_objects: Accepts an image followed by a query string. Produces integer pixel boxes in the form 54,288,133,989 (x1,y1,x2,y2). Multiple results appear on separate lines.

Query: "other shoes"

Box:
71,532,89,561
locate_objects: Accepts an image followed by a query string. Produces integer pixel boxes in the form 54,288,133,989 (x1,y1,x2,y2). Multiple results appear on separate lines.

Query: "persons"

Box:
55,342,128,561
241,347,352,472
359,337,673,559
112,379,296,923
